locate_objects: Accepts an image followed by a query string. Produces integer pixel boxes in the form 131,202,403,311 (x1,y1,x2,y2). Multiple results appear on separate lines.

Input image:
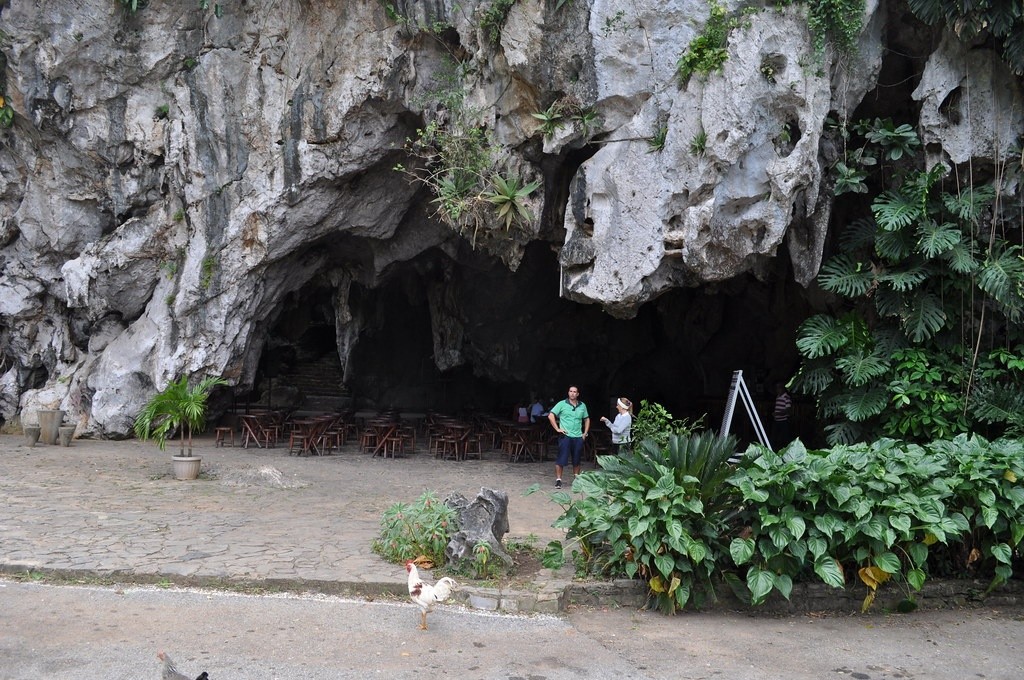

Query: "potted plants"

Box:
135,374,230,479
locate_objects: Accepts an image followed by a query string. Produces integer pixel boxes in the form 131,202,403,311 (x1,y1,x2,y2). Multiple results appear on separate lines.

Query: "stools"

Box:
215,427,234,447
239,407,611,469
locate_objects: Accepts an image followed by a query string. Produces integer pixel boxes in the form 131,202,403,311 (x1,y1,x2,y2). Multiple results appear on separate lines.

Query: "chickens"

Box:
405,559,458,630
156,651,208,680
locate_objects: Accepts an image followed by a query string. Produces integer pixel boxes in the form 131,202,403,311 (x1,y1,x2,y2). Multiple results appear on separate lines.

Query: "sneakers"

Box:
555,478,562,489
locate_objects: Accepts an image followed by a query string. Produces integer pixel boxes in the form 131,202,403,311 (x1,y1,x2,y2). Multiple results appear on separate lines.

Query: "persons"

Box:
548,379,591,488
513,402,520,421
601,398,633,457
527,402,544,423
773,383,792,423
517,407,529,422
